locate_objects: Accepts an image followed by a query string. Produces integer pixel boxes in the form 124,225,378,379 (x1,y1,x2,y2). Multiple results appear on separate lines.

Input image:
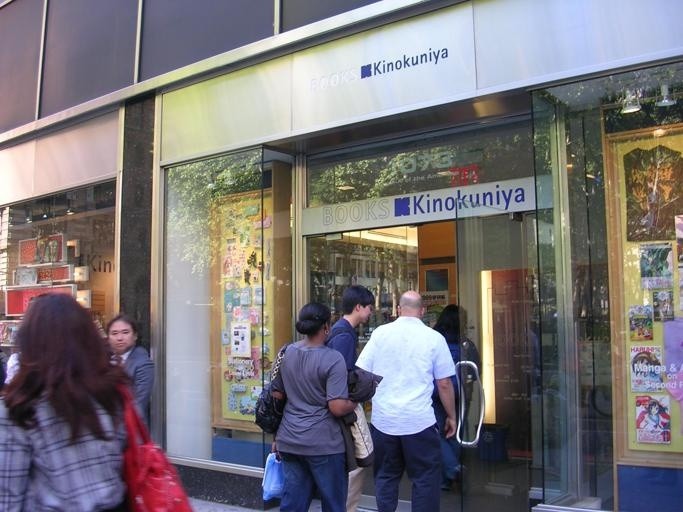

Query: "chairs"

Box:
440,464,469,495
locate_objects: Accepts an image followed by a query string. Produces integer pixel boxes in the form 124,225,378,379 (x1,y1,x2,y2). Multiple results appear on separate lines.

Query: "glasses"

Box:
620,87,642,114
653,84,675,106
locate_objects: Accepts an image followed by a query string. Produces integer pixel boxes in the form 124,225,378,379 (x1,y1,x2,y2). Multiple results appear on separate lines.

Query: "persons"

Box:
324,283,377,511
431,300,480,494
1,290,144,510
355,290,460,512
270,301,362,512
104,314,156,444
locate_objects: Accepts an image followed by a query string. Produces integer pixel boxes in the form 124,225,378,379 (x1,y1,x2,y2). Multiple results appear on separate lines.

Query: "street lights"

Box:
122,443,194,512
255,373,286,432
